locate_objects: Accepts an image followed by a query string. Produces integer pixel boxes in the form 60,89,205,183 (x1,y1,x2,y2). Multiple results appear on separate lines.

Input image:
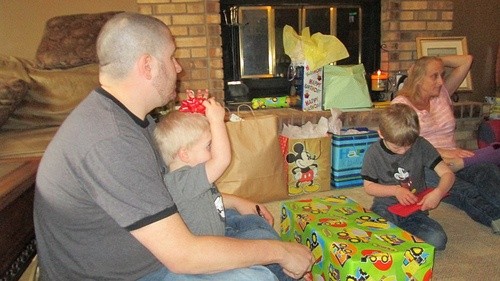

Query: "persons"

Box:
32,13,316,281
392,54,500,234
152,97,283,280
361,103,455,250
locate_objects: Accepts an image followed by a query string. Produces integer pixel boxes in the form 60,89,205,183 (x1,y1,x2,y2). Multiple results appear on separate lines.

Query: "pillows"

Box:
0,56,101,132
0,56,33,127
35,11,125,70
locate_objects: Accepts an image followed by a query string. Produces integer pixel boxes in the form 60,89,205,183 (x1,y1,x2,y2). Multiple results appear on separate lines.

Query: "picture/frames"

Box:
415,35,473,93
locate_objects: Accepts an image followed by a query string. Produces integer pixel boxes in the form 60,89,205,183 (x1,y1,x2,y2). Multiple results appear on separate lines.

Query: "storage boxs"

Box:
289,61,323,112
328,127,380,190
280,193,435,281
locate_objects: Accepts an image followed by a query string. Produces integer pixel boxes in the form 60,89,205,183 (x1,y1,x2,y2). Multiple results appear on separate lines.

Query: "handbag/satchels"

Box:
321,64,374,110
211,104,290,204
279,131,333,196
328,127,380,188
287,59,324,112
